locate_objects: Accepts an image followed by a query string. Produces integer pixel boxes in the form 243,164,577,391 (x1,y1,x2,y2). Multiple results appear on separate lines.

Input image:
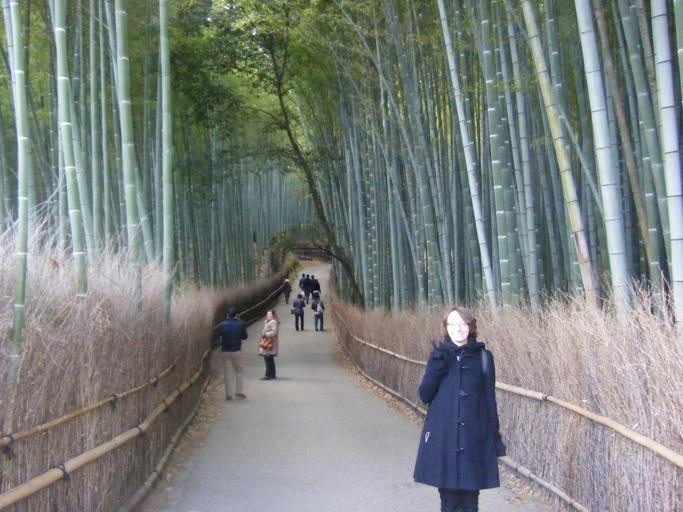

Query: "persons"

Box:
258,308,280,380
412,307,507,512
215,307,248,401
282,273,325,331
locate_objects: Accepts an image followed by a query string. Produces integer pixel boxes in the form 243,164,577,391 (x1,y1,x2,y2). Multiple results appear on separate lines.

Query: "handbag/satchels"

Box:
259,337,273,351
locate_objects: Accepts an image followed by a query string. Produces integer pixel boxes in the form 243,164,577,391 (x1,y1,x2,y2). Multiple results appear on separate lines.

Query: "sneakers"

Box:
297,328,324,331
260,375,276,380
227,393,246,400
